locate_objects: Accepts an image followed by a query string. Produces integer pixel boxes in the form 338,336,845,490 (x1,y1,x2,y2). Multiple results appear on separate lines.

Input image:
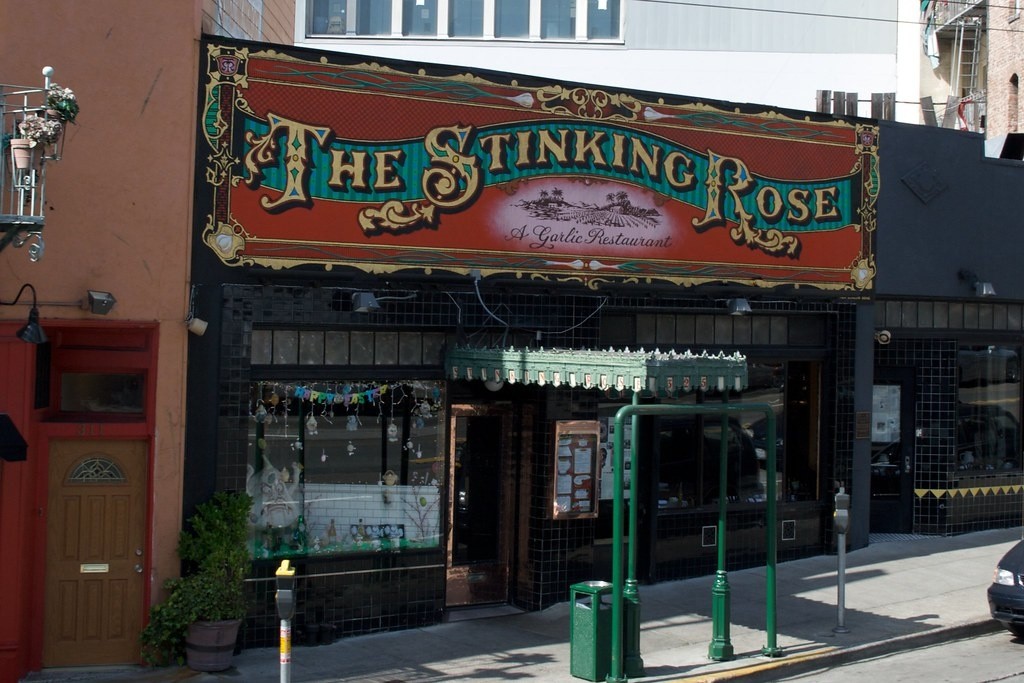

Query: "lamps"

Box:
726,298,752,317
86,289,117,315
0,284,46,343
351,291,417,313
957,269,996,298
185,283,208,336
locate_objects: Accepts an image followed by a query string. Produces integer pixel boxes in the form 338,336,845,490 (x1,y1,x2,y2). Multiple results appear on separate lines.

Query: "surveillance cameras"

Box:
878,330,890,344
189,318,206,335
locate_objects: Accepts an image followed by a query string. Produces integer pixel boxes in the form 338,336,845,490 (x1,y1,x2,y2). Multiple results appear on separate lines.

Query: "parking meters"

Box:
275,560,298,683
833,486,852,633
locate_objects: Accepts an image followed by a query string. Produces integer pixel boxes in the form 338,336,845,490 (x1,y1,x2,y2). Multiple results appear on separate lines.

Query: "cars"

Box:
987,540,1024,635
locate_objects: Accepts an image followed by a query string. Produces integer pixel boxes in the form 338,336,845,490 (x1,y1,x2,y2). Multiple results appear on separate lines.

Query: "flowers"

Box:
18,115,61,148
47,82,78,120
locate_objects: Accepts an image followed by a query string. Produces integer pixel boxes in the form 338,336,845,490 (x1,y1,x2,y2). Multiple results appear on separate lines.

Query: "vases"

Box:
44,109,64,122
10,138,44,169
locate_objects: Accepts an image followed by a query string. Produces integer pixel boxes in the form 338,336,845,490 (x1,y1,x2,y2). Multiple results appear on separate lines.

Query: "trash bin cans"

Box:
570,581,613,682
